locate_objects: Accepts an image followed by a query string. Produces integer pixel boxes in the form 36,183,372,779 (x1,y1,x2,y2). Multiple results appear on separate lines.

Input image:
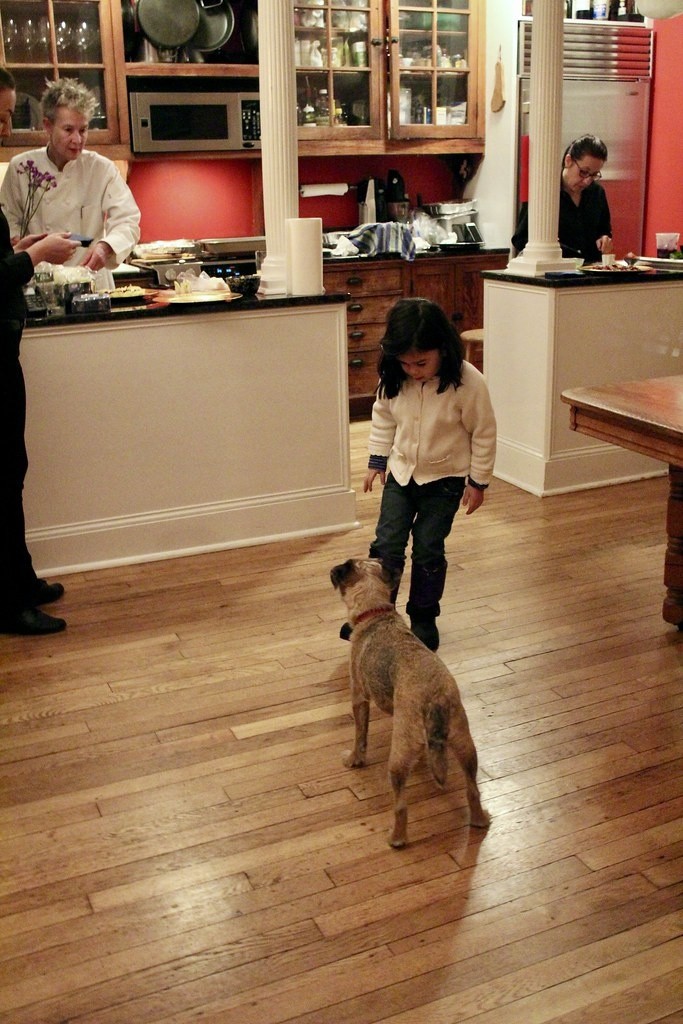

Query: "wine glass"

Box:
0,14,100,64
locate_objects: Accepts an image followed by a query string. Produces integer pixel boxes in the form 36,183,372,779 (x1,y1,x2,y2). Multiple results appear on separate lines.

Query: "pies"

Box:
96,286,146,297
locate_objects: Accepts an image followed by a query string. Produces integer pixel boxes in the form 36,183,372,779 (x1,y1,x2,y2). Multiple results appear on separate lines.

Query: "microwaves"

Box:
129,92,262,153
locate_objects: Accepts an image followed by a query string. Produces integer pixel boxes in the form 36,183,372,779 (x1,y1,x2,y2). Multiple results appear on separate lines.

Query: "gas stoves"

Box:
128,252,258,286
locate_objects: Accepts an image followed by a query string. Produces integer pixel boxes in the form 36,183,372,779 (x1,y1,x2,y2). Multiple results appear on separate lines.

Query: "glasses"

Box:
576,161,602,181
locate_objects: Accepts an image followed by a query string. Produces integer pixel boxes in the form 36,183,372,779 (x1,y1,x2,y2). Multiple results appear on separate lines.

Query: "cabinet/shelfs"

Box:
0,0,484,156
321,255,508,406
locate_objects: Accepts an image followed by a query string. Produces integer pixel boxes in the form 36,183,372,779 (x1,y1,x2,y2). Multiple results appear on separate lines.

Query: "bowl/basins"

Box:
225,275,261,297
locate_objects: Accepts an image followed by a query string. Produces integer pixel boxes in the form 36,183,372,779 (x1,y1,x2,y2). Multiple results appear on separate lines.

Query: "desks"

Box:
559,374,683,632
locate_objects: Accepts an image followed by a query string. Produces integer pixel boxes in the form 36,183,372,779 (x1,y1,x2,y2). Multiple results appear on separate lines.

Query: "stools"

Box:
460,326,482,369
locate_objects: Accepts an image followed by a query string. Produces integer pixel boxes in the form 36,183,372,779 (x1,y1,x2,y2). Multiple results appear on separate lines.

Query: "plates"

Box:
577,265,653,275
153,289,243,304
31,234,94,248
11,92,43,130
111,288,159,306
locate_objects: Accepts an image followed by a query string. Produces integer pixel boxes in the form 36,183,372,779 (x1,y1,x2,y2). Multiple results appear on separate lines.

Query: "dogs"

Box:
330,557,493,847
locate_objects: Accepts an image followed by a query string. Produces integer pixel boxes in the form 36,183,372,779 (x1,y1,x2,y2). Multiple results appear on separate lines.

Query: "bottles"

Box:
617,0,626,16
33,261,57,309
297,87,370,128
591,0,608,20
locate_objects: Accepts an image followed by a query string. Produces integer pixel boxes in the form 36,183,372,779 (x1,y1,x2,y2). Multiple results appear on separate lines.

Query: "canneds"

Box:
353,51,368,66
414,104,431,124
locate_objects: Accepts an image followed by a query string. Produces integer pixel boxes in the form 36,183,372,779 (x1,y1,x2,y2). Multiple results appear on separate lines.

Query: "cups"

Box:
602,254,615,268
576,0,592,20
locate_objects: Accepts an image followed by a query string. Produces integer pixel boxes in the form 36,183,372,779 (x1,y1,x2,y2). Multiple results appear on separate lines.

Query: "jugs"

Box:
655,232,680,259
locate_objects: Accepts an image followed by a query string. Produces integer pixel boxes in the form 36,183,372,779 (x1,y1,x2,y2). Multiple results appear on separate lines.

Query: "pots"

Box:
136,0,235,58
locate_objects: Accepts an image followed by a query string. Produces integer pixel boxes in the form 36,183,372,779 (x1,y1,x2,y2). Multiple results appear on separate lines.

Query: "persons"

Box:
0,76,142,272
340,298,499,656
511,133,612,268
0,67,71,636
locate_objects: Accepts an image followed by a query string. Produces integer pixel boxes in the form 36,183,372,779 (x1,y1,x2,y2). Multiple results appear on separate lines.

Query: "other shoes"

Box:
1,611,66,636
409,620,440,653
35,582,66,604
340,624,352,642
